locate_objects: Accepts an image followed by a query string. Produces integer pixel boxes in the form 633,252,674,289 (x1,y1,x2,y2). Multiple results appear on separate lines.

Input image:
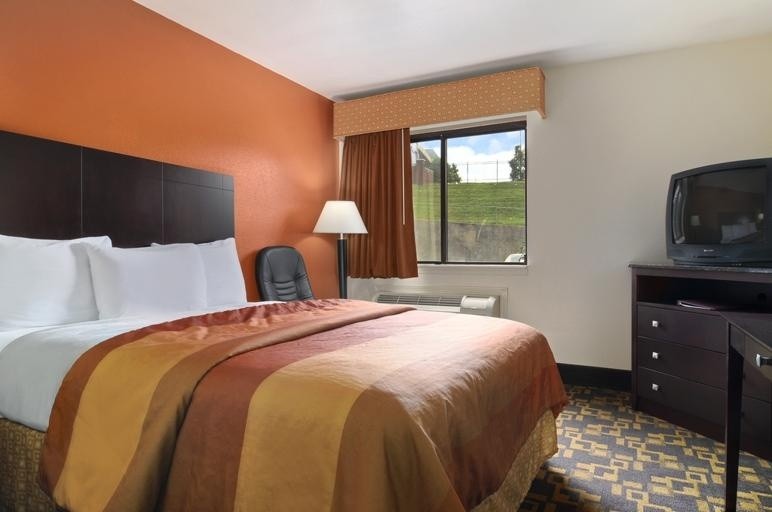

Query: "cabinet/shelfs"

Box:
628,263,772,462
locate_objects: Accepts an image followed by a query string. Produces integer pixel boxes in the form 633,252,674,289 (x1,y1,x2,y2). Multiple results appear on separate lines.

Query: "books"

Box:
676,296,738,311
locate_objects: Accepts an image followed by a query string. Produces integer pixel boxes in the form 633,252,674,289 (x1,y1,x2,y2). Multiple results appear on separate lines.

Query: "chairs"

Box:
256,246,315,301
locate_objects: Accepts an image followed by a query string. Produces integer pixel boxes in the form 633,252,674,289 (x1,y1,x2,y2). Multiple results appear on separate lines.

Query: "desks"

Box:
718,312,772,512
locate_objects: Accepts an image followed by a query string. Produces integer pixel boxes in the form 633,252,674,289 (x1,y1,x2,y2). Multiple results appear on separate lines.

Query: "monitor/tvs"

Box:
664,157,772,268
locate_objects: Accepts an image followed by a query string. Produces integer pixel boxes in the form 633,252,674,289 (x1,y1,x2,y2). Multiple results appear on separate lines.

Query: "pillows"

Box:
0,235,247,331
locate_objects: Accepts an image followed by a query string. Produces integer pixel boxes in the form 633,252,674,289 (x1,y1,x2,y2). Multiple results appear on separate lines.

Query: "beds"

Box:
0,130,570,512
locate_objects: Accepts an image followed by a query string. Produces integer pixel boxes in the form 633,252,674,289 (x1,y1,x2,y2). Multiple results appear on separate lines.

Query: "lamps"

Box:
312,200,368,299
